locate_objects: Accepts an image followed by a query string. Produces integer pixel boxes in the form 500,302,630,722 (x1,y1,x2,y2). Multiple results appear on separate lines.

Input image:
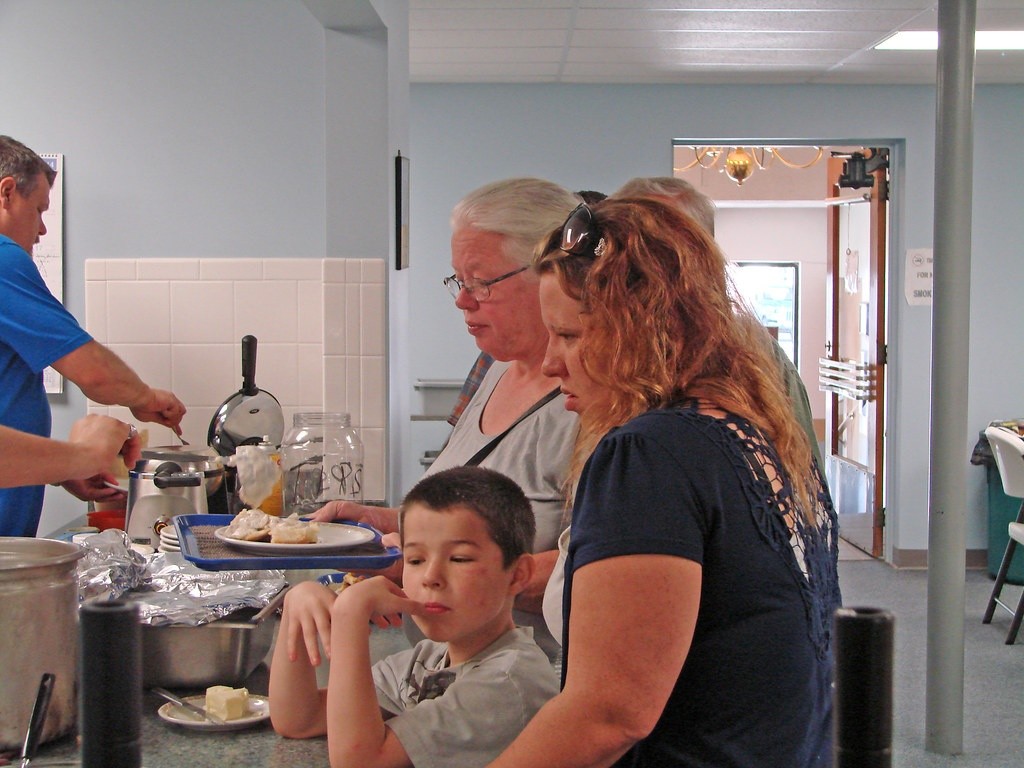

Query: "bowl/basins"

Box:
87,511,125,533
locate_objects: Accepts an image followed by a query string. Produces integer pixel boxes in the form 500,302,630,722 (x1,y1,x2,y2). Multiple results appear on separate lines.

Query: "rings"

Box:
128,424,137,439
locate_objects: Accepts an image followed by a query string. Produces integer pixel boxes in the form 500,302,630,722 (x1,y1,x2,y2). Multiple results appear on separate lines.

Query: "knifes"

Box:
152,688,227,725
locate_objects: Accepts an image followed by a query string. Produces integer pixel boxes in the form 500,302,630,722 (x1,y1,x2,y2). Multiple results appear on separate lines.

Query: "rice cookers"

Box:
124,446,240,550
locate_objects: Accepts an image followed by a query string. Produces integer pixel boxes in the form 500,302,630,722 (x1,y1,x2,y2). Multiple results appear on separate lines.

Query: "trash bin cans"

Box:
979,424,1024,584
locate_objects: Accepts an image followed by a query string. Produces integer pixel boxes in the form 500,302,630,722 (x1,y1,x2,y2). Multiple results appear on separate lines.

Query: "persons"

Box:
299,174,845,767
0,413,143,503
0,134,187,538
268,465,560,768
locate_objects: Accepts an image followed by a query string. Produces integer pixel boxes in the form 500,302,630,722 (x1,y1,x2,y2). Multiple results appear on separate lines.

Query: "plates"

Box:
131,543,155,555
158,695,271,731
215,520,375,555
104,478,129,492
157,526,182,555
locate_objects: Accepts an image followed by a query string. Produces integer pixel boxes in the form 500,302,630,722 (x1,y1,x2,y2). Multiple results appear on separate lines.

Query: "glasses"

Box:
559,202,608,259
444,264,533,301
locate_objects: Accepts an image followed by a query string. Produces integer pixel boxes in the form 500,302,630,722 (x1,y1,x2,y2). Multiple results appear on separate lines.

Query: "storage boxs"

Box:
78,577,296,689
418,448,439,474
413,375,466,416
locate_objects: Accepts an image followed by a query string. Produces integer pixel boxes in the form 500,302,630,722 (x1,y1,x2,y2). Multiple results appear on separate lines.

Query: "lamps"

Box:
673,146,830,188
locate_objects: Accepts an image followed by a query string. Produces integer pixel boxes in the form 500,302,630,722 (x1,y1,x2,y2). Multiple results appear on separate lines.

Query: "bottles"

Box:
280,412,363,522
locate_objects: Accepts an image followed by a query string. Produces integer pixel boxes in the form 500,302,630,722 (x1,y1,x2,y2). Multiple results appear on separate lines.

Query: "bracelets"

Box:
51,483,60,486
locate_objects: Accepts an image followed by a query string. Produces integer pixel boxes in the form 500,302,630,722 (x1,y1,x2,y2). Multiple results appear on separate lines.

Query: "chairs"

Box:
983,424,1024,646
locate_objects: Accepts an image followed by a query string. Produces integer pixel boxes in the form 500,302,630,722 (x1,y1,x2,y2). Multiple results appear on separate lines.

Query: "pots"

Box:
0,535,88,752
